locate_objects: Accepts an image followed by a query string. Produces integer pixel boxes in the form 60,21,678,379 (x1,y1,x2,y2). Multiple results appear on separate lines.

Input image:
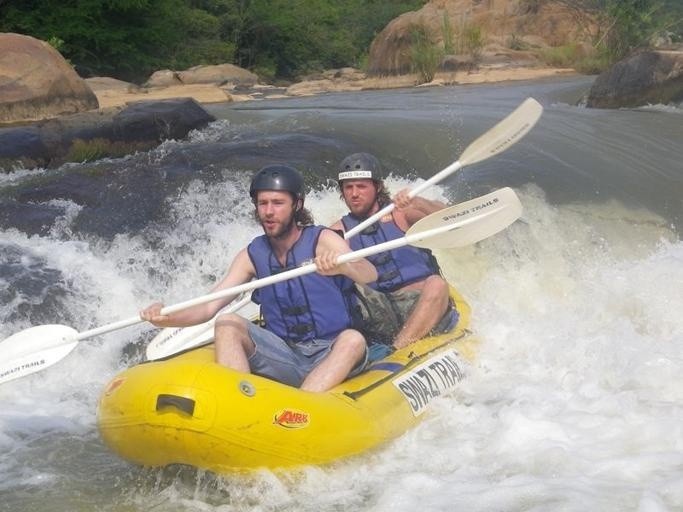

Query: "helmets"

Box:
249,165,305,197
337,152,382,183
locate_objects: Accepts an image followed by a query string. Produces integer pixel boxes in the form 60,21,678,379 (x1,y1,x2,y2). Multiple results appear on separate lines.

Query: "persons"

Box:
328,153,458,362
140,164,378,393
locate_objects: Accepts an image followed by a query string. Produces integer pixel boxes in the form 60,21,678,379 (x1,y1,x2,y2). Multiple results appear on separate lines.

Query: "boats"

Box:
98,280,479,469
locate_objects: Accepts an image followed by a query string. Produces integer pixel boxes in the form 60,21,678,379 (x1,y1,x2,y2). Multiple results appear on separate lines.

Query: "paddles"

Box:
0,182,526,383
144,94,545,367
